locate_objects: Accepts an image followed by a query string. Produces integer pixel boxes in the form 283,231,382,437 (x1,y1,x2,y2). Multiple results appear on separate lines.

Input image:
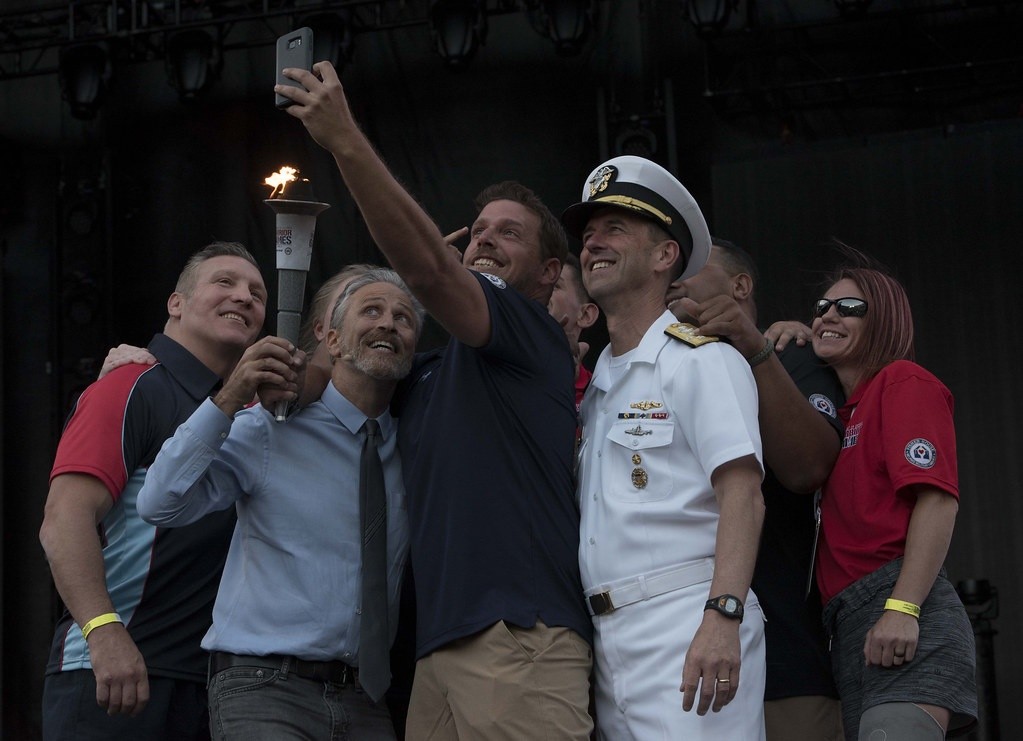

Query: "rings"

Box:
893,652,906,659
716,677,731,682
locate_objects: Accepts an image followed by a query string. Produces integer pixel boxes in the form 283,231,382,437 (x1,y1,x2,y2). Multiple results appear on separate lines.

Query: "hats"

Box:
559,155,712,281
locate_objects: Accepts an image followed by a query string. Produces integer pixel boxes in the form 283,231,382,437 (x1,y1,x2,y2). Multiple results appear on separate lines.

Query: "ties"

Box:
358,419,392,703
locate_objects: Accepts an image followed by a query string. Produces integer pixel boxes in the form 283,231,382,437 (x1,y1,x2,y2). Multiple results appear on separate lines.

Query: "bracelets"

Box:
746,337,773,368
886,597,922,617
84,613,125,640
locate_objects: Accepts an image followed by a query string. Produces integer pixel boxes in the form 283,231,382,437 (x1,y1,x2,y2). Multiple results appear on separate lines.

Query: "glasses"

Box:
812,298,869,317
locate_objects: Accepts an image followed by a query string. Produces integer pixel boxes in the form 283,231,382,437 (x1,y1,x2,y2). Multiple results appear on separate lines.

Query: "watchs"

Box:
701,593,745,625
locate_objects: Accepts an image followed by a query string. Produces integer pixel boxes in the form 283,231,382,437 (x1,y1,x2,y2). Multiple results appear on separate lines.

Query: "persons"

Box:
561,155,769,741
274,61,601,741
98,242,982,741
38,238,270,741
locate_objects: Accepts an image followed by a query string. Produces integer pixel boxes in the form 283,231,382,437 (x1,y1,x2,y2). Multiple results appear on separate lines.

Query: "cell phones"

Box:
275,27,315,110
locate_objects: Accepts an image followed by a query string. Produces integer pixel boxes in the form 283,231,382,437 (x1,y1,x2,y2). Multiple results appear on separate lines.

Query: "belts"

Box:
209,650,356,685
585,559,715,616
